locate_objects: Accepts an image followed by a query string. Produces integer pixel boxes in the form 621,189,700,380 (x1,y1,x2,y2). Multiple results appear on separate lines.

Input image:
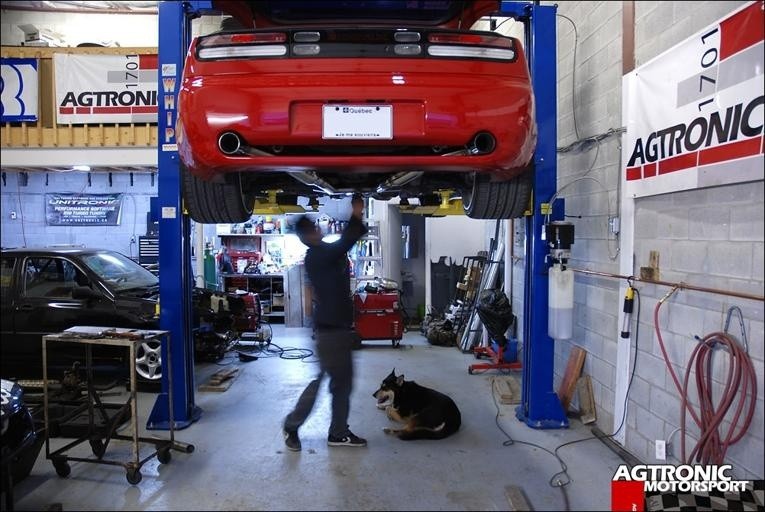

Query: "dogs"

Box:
372,368,460,440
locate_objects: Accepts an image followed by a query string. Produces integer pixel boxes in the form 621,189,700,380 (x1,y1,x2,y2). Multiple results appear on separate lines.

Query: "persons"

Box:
282,196,369,451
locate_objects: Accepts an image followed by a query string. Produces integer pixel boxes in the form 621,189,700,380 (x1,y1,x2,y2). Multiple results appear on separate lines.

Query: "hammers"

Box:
102,332,144,341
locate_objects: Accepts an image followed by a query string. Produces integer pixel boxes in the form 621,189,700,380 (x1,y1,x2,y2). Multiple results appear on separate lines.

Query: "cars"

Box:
174,3,540,228
0,244,242,397
1,378,39,496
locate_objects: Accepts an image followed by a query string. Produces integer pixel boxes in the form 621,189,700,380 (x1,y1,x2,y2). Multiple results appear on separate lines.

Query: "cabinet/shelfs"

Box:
220,272,289,327
355,219,383,291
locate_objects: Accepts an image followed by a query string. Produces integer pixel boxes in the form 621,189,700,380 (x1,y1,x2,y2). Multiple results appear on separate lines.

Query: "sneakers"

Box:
281,418,301,451
328,431,367,447
